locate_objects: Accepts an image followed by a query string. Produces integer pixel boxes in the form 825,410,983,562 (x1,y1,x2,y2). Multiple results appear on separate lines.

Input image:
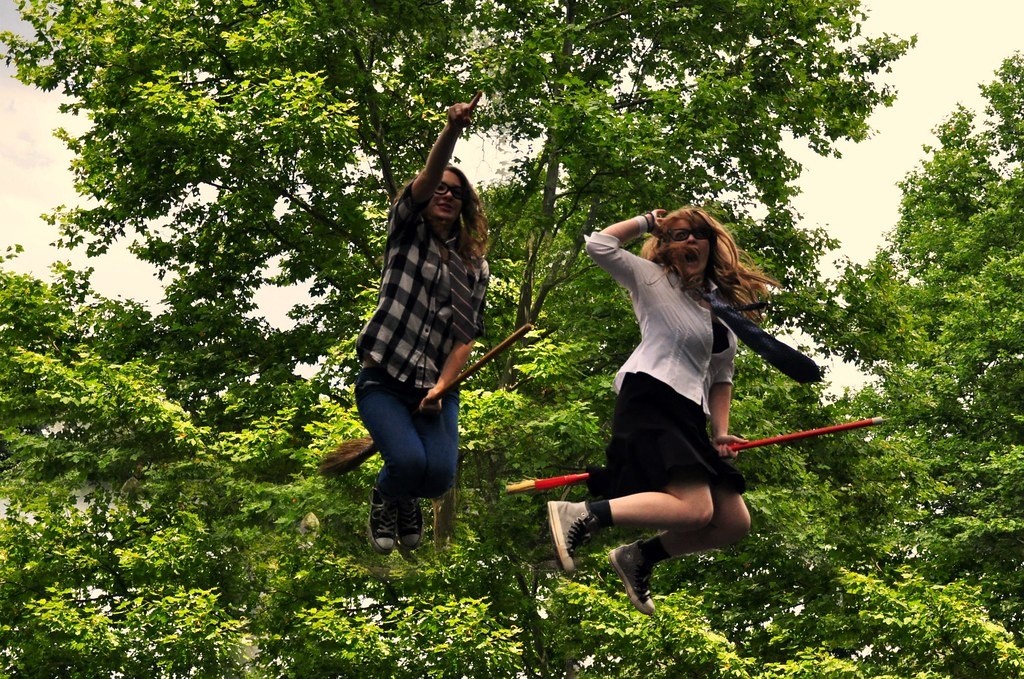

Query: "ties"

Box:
693,283,822,383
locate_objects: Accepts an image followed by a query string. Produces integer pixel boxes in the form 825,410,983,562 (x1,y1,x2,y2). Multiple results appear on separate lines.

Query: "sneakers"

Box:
396,494,422,547
608,538,655,614
547,497,600,570
368,479,397,555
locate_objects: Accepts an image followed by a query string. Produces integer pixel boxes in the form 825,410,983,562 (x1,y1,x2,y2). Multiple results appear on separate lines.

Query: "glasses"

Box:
435,181,465,200
667,228,711,241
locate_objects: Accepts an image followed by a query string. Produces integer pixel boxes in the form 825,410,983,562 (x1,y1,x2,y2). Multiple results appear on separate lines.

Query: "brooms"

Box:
315,323,533,478
505,416,883,495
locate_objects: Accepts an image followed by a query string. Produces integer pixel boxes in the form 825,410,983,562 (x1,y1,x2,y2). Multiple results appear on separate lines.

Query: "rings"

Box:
456,109,461,111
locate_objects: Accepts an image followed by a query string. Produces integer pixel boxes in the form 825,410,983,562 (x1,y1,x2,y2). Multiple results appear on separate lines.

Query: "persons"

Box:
548,207,823,614
354,87,489,554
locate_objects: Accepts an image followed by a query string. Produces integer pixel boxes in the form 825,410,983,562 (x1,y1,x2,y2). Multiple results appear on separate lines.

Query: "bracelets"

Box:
642,211,656,233
